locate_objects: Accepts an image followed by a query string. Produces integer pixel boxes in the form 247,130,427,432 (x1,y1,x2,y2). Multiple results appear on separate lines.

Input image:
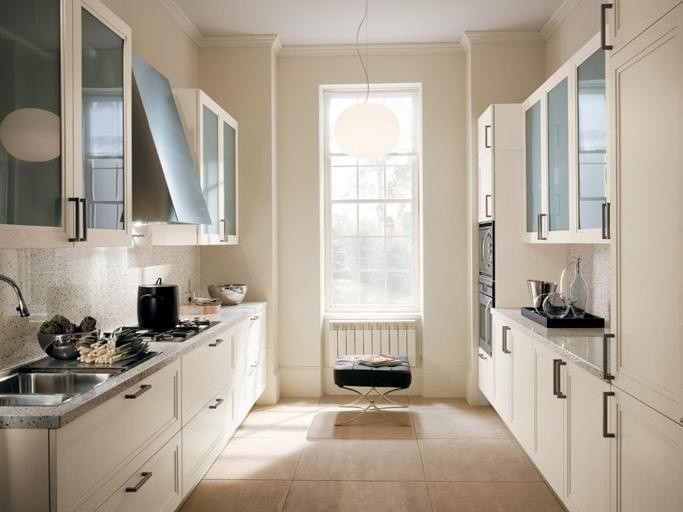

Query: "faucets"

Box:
0,273,30,317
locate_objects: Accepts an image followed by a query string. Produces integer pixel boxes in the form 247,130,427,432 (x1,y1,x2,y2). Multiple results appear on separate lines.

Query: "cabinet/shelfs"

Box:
65,424,182,508
531,330,620,507
486,306,536,453
609,3,683,507
232,302,269,384
181,318,257,422
575,27,613,245
182,383,242,496
234,353,285,431
149,87,242,248
1,1,134,251
476,103,530,401
527,60,575,245
49,363,187,507
602,1,683,60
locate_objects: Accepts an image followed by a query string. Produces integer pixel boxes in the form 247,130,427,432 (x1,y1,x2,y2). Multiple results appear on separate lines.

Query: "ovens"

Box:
477,221,495,283
477,281,494,356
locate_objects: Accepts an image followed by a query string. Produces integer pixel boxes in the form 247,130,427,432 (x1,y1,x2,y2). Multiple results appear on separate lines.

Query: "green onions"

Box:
73,327,150,364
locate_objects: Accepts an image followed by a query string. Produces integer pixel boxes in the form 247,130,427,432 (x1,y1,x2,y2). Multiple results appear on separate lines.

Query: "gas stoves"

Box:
117,317,221,343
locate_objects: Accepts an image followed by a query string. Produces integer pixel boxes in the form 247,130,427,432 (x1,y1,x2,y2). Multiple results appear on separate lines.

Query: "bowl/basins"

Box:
208,282,246,305
36,329,101,361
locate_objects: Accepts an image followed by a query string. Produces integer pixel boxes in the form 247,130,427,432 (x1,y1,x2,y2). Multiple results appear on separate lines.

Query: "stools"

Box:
332,353,412,428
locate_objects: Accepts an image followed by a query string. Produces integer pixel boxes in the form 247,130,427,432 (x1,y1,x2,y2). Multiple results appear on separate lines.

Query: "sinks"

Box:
0,394,79,407
0,371,120,396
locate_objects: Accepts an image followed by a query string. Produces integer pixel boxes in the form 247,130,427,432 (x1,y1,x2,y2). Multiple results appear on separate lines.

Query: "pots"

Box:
137,277,180,332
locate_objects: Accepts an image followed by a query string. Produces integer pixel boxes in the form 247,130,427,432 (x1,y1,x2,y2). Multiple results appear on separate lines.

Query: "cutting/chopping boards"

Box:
18,350,158,373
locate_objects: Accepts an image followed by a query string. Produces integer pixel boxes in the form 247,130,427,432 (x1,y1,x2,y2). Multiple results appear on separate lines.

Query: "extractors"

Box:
133,51,213,225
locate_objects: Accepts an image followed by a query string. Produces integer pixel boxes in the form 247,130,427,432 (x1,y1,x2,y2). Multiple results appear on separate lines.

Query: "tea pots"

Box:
560,257,588,317
534,292,572,319
